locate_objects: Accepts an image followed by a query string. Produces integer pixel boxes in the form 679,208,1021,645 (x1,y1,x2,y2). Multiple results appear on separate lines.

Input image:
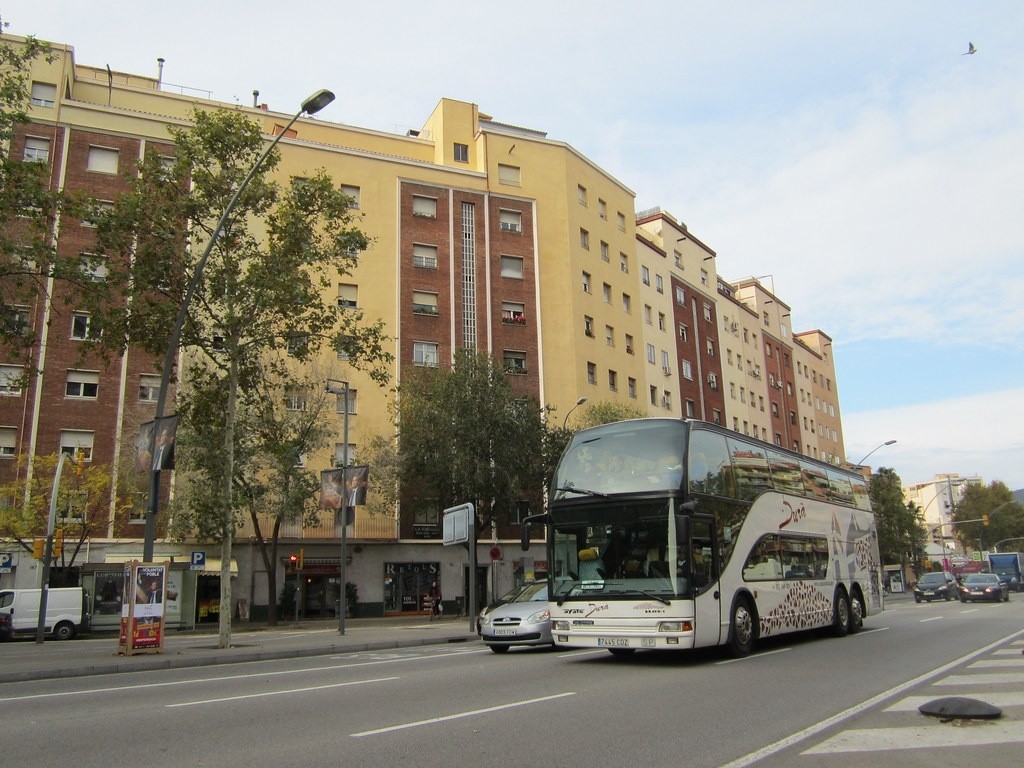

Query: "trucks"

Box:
988,552,1024,593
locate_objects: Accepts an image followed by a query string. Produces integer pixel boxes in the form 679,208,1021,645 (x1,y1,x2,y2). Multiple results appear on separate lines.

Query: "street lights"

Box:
142,89,337,565
852,440,898,474
562,397,587,429
919,477,968,526
979,499,1016,565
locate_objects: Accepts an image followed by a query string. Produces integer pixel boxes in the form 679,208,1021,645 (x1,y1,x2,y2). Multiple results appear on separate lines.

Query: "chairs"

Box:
577,548,607,581
622,450,708,491
626,558,670,580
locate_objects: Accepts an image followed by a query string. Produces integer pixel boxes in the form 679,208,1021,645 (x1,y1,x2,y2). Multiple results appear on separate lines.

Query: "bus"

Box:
519,417,884,663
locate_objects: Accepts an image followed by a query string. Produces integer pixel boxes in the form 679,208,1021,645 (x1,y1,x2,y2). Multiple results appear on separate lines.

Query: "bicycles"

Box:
427,596,443,622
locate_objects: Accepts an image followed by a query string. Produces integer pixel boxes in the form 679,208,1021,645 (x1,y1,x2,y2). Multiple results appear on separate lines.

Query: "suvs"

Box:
913,571,960,602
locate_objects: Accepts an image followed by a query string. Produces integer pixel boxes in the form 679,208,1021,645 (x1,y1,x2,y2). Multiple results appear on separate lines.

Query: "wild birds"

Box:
961,42,978,56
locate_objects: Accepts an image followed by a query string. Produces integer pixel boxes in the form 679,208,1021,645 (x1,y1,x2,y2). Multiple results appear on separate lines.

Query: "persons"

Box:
124,582,129,604
426,580,441,621
146,581,162,603
99,575,120,603
348,472,365,506
667,545,696,576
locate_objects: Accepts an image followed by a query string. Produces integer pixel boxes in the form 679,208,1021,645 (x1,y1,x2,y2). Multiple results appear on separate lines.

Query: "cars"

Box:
960,573,1009,603
477,581,534,637
479,575,573,654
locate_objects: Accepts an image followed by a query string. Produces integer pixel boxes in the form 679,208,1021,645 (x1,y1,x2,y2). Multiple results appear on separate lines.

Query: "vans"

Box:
0,587,92,640
